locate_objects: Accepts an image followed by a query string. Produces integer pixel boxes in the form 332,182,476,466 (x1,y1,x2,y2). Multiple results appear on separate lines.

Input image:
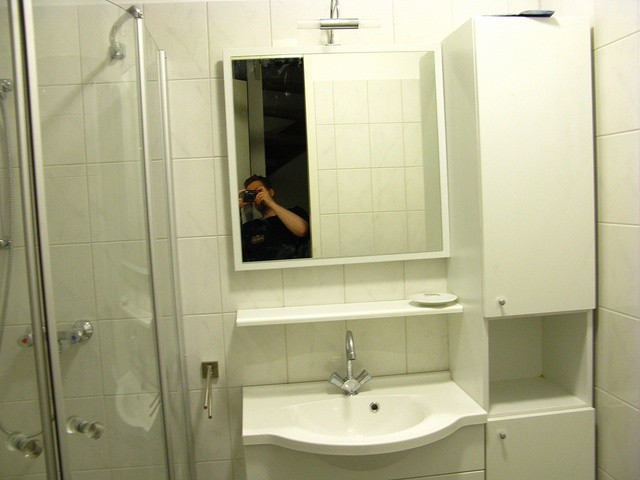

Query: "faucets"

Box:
329,331,371,393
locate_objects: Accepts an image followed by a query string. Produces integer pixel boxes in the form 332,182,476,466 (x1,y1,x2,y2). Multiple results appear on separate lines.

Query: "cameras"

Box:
243,190,262,202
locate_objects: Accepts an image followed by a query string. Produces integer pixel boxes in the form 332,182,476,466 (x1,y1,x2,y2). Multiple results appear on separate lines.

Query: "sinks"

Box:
285,394,431,439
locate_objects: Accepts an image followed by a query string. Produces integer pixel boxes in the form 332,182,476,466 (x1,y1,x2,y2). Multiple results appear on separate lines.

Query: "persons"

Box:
237,175,308,263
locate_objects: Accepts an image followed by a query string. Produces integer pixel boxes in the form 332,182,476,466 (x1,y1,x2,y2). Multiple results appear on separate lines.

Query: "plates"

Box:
411,293,458,308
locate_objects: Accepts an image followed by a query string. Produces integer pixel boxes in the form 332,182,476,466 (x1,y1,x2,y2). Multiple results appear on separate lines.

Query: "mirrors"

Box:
221,42,451,272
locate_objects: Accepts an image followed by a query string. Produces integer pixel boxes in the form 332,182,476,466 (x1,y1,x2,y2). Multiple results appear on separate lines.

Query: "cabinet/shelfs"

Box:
236,18,597,480
